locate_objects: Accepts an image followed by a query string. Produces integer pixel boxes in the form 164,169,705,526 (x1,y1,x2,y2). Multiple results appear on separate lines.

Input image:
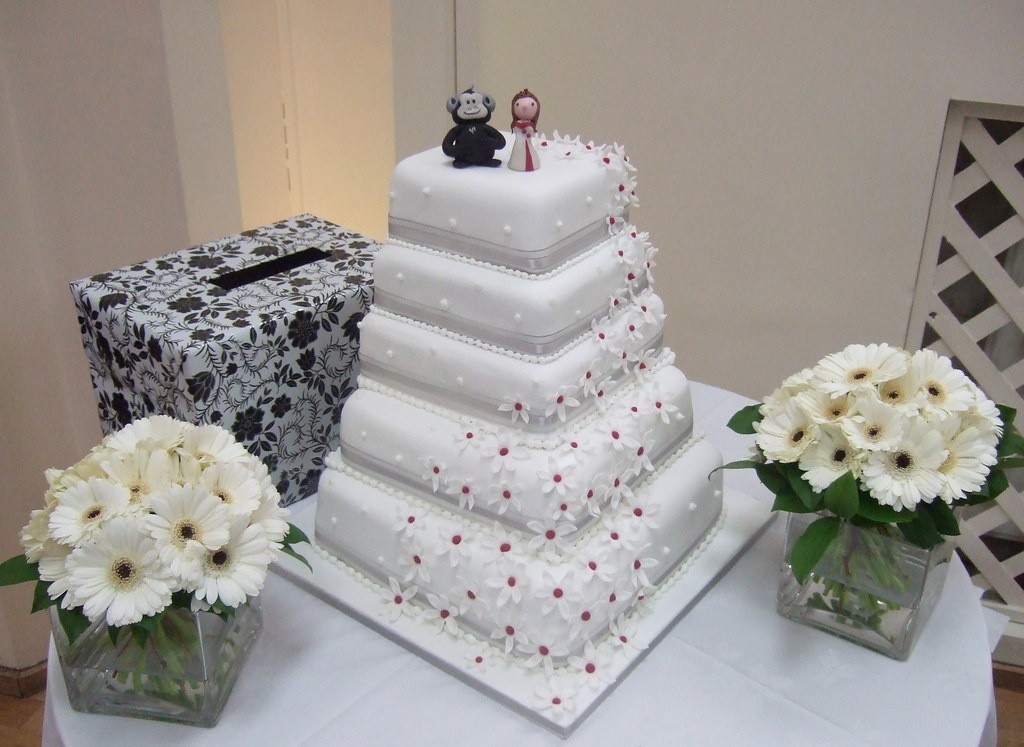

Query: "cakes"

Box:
314,128,725,677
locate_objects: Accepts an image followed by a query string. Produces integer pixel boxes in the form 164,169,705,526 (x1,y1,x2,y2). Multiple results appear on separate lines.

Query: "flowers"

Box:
707,342,1024,633
0,415,313,709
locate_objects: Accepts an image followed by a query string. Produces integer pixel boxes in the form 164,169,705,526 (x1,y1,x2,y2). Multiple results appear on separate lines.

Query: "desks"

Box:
44,377,998,747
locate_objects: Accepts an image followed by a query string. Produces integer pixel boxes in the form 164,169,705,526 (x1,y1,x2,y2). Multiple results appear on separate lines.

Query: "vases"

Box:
776,510,956,661
49,592,262,728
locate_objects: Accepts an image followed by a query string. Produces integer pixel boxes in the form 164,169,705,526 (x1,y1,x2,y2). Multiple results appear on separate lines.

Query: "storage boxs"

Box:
68,214,378,508
